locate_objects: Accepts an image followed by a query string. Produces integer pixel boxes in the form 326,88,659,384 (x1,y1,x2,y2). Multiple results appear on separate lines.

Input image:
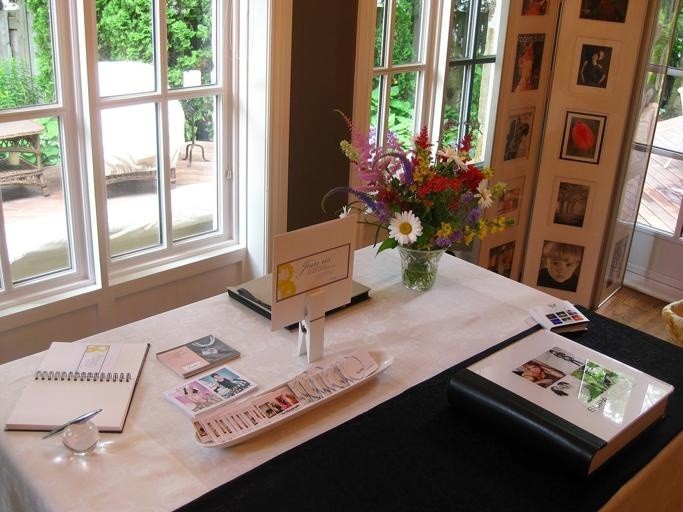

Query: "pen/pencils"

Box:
42,408,104,438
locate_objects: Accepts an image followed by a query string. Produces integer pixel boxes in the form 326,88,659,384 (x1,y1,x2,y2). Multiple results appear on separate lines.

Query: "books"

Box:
162,365,258,420
4,340,153,436
530,301,590,336
155,333,240,379
447,330,675,476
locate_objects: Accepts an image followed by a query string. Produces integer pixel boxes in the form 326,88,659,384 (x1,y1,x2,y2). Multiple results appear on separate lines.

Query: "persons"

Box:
580,53,605,85
542,241,581,291
558,382,574,390
209,372,250,396
549,349,582,369
519,363,563,387
593,47,607,70
181,388,210,407
517,38,537,95
550,385,567,399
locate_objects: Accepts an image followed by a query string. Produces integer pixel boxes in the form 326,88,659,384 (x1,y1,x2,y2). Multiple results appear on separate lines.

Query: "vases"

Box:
394,244,448,294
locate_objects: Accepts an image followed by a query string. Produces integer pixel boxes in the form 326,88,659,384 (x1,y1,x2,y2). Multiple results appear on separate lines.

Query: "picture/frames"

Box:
555,105,610,169
564,32,621,96
544,172,599,234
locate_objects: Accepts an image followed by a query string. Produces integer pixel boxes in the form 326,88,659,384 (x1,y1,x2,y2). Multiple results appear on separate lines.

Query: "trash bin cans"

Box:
662,298,683,347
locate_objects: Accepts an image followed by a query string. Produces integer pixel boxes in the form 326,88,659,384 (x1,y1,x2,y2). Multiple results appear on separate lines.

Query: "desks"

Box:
1,236,683,511
0,117,51,203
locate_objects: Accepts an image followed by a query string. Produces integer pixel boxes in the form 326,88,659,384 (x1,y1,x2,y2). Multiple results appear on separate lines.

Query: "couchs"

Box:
94,54,186,190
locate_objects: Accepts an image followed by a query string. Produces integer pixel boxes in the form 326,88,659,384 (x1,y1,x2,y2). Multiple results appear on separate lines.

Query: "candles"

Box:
180,68,201,89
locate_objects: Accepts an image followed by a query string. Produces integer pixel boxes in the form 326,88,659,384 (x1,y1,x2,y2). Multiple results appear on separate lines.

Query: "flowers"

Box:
322,105,514,257
